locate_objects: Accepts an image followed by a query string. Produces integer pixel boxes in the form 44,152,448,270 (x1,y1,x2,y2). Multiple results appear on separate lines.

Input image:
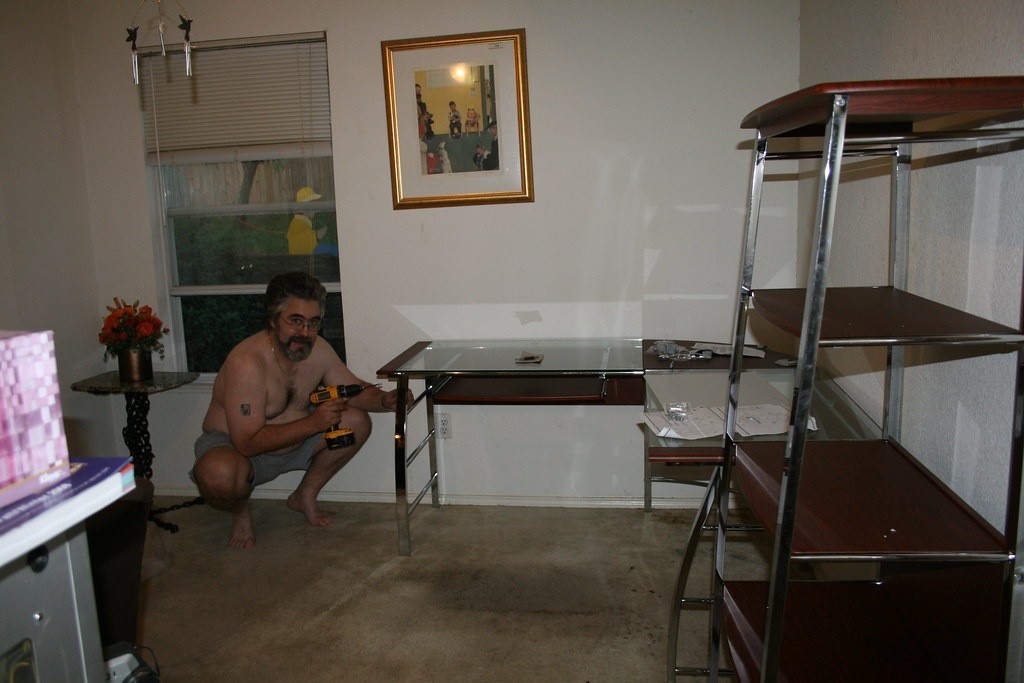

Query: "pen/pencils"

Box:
515,356,539,360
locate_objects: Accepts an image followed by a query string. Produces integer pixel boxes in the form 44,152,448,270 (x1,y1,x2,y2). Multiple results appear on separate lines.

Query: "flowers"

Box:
98,297,170,360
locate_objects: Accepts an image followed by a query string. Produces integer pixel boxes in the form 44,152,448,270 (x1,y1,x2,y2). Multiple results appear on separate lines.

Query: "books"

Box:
0,454,139,566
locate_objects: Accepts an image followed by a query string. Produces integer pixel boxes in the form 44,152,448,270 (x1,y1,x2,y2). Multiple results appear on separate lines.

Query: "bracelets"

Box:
265,328,301,376
380,394,392,409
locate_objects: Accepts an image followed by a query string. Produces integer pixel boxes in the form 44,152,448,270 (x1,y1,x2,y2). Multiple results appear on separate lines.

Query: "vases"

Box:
116,349,154,382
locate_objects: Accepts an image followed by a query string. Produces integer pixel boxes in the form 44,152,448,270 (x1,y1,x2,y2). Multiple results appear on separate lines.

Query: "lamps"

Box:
125,0,194,85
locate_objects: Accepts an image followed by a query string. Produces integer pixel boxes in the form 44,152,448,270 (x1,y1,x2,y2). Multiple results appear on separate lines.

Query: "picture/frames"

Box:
380,28,535,211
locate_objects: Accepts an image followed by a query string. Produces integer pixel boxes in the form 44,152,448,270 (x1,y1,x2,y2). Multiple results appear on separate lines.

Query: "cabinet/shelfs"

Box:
663,74,1024,683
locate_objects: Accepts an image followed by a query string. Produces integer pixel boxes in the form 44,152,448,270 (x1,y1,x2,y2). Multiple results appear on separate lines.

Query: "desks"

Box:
376,340,811,556
71,370,207,534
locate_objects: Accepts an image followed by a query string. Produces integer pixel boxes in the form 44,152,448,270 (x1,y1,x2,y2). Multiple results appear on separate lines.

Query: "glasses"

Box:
279,315,325,332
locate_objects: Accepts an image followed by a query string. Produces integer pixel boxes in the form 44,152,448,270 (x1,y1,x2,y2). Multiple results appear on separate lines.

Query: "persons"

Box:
187,271,414,548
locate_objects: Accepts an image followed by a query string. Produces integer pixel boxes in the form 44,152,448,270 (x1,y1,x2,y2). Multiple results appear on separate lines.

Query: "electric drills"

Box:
309,383,383,451
